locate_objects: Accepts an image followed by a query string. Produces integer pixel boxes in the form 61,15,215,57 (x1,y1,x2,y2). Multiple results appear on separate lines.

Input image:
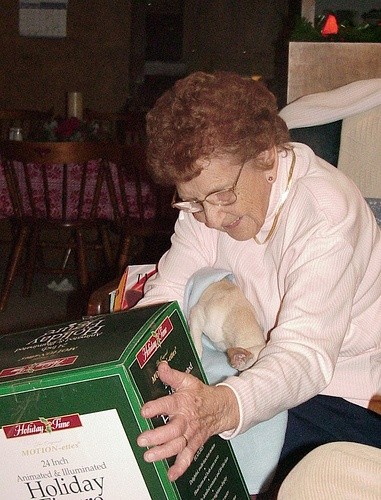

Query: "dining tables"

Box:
0,157,182,220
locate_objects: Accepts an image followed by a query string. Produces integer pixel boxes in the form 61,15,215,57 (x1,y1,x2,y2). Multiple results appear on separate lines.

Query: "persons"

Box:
110,71,380,481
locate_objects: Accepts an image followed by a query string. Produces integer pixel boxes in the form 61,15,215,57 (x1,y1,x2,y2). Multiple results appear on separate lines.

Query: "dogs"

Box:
188,277,267,374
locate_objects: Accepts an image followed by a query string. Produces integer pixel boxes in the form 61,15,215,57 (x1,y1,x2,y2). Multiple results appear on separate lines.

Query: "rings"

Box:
180,432,191,450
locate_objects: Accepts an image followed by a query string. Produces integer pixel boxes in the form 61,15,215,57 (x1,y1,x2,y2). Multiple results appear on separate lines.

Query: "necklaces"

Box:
252,144,296,246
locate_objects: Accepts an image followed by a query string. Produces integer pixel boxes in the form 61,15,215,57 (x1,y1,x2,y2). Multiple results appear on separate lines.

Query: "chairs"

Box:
0,139,118,318
101,139,183,280
0,109,61,146
114,116,148,147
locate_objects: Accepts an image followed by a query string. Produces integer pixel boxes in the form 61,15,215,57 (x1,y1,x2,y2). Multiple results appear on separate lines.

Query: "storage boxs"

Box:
0,299,252,500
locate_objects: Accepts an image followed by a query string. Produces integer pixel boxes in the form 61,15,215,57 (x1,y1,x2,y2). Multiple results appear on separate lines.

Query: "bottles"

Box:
9,126,24,141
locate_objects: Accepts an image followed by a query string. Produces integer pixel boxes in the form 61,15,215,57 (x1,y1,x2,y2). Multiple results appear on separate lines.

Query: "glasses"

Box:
171,157,247,212
